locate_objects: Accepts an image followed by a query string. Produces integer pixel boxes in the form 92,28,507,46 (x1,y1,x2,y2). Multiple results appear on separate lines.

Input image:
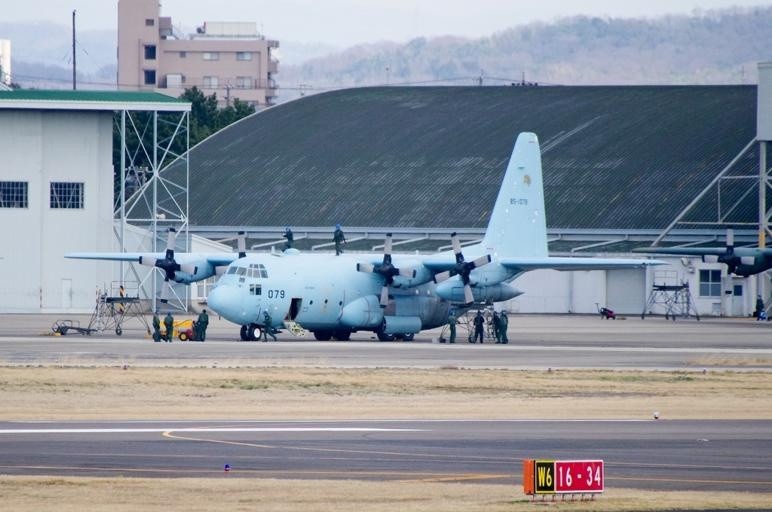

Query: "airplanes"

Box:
63,133,671,340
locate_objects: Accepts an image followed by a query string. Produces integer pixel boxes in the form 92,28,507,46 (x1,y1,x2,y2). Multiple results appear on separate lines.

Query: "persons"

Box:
473,310,485,344
332,224,347,256
281,227,294,252
153,311,160,342
489,310,509,344
262,310,276,342
755,295,765,320
448,312,460,343
196,310,208,341
164,312,173,342
